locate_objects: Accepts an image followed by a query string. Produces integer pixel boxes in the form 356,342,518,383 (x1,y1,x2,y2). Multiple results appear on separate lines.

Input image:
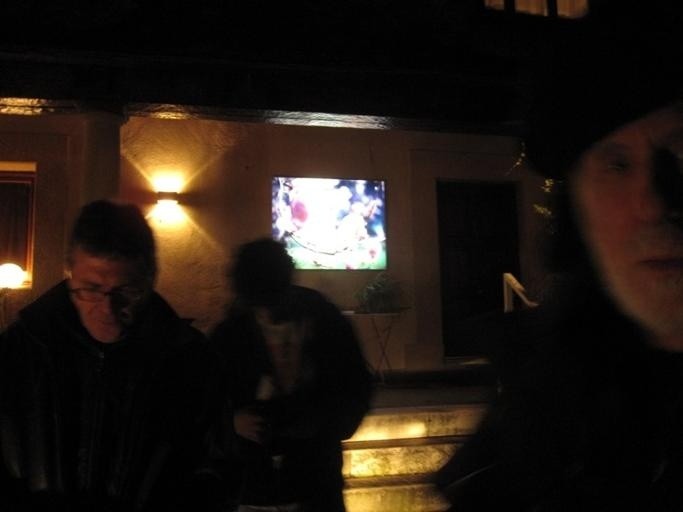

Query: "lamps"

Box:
155,191,181,206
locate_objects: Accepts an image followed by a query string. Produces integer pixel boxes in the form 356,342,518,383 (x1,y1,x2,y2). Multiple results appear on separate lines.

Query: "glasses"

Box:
66,288,151,304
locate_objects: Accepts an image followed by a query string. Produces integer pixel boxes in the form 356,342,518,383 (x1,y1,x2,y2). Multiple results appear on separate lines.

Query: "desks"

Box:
340,309,401,385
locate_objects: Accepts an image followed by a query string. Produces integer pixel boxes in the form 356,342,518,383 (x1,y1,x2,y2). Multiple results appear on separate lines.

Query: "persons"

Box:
429,1,682,509
0,198,223,512
199,235,373,511
